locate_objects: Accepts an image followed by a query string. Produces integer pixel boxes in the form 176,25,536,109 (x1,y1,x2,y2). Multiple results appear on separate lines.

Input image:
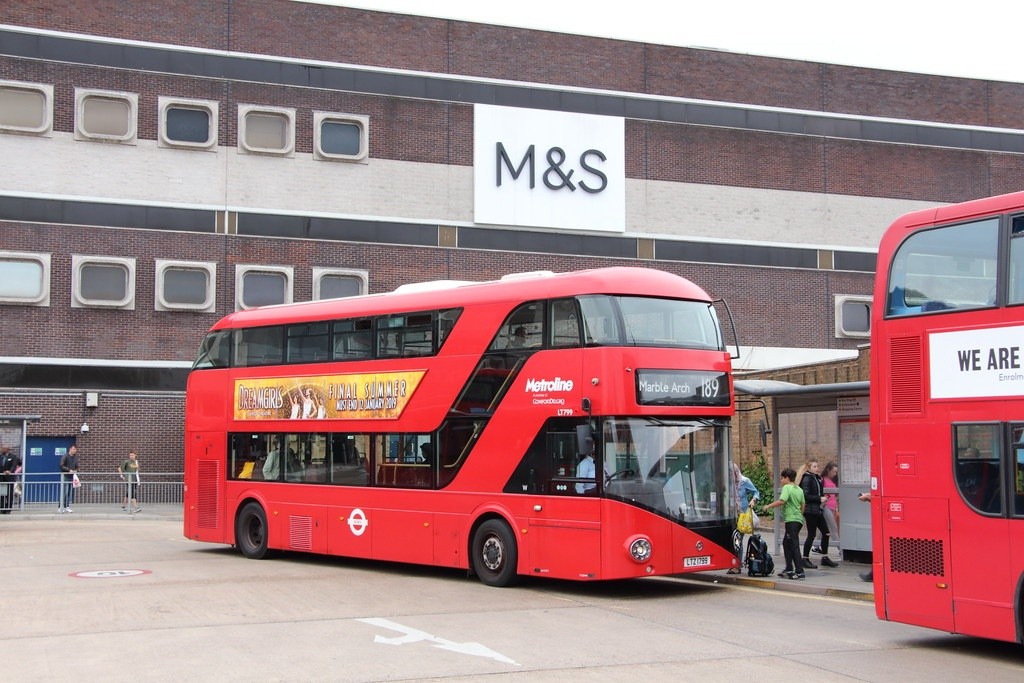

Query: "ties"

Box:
593,460,610,478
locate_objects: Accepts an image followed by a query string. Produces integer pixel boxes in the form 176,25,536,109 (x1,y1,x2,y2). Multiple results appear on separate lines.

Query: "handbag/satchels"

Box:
73,473,81,488
738,507,760,534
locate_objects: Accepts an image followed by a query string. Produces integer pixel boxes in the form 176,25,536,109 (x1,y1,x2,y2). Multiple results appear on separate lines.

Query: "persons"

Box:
286,380,329,420
964,448,980,460
0,445,22,514
733,458,875,583
575,442,617,497
506,327,531,348
118,450,142,512
262,435,434,490
58,444,79,512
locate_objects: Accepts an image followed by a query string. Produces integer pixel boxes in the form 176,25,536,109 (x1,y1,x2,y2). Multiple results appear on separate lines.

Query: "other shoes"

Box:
726,568,741,574
858,570,873,582
134,507,142,513
812,547,821,553
65,507,73,512
58,508,64,512
121,506,127,512
802,558,818,569
821,556,838,567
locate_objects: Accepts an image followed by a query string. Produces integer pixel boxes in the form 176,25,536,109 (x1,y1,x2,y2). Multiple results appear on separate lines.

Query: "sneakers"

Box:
778,570,793,577
789,572,805,580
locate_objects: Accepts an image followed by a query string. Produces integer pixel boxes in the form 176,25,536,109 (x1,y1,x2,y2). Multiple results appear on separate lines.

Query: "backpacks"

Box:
745,534,774,576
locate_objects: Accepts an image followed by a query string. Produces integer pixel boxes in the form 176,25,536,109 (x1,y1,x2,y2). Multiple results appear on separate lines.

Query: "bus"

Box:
867,190,1024,645
183,266,775,589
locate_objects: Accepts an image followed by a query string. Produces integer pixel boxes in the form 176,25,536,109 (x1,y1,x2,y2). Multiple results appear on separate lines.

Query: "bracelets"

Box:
120,475,124,478
